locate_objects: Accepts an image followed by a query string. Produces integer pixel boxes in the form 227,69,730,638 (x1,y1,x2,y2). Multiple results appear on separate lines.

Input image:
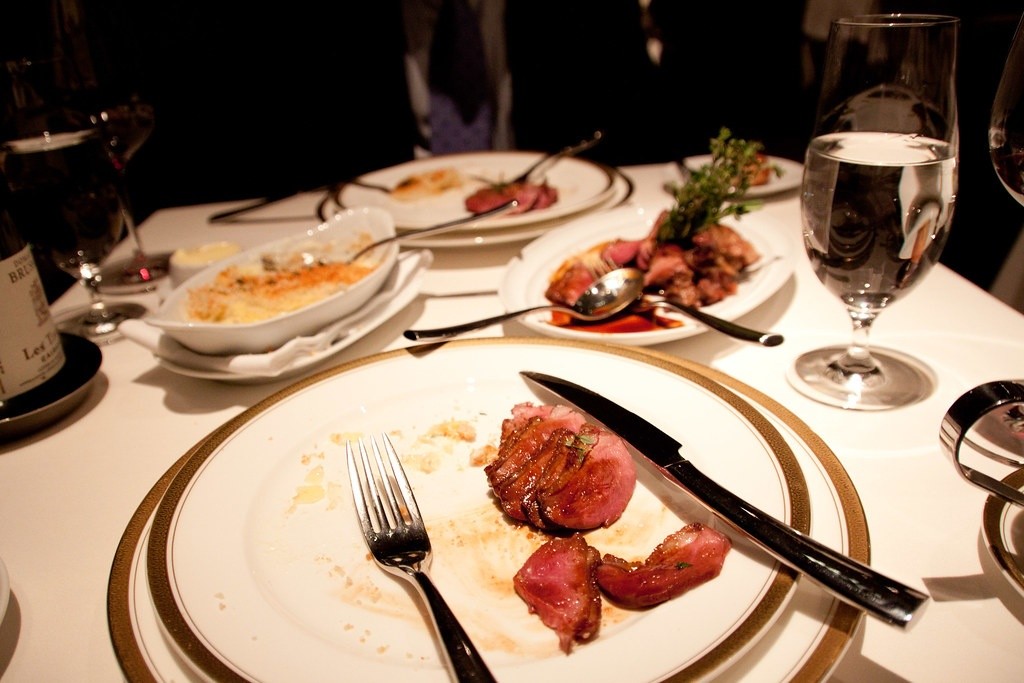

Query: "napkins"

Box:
116,247,434,379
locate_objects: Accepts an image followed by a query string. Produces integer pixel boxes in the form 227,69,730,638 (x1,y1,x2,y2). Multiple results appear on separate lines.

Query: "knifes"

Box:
519,369,930,632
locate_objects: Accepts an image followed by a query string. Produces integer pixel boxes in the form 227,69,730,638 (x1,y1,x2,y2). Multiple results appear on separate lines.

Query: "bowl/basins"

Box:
144,205,399,352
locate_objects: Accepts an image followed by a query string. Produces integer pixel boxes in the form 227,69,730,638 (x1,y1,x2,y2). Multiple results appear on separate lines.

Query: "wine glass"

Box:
964,20,1024,465
43,45,173,295
784,12,961,410
8,130,148,348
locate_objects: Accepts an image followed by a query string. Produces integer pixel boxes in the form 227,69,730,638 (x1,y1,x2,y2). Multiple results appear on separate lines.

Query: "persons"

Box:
501,1,810,168
75,1,413,243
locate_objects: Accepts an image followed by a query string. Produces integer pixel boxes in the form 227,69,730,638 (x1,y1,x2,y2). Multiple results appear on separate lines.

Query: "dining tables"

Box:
0,159,1024,683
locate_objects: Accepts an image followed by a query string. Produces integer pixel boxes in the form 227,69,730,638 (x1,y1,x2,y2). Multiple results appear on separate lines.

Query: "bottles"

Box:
1,176,71,421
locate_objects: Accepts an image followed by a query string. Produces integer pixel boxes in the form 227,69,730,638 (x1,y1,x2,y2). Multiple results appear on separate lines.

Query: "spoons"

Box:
402,266,644,339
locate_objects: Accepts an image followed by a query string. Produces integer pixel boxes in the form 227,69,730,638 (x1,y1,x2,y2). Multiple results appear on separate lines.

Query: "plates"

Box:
667,154,804,197
978,466,1024,598
145,340,813,683
497,204,795,345
332,152,613,233
316,165,635,248
106,337,872,682
0,329,104,434
158,263,427,383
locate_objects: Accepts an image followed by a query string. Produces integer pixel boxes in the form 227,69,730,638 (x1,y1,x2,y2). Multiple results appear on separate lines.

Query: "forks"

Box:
344,431,499,683
587,256,782,348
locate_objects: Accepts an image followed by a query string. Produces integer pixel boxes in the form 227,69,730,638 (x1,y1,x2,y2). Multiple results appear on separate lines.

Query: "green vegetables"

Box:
660,127,784,249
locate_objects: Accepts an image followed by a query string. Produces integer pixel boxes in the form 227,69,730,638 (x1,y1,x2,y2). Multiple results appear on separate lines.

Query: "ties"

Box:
427,1,499,154
